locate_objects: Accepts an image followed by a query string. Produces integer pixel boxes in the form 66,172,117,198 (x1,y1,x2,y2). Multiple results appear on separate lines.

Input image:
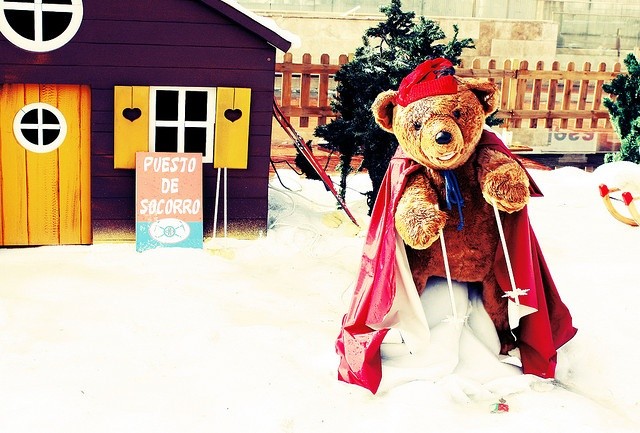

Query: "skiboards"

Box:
273,95,360,228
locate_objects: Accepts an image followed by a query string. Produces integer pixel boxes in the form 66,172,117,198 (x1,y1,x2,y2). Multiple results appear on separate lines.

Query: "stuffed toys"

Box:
371,56,528,383
